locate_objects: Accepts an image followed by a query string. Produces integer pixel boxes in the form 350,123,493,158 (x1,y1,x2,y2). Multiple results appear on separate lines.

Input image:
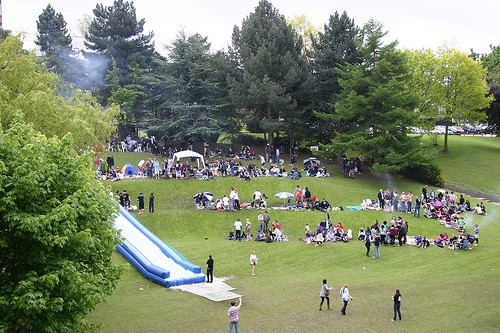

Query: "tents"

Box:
120,163,137,175
172,150,205,170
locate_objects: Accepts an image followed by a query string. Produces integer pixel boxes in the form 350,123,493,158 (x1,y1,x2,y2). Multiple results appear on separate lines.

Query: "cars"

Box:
404,116,498,136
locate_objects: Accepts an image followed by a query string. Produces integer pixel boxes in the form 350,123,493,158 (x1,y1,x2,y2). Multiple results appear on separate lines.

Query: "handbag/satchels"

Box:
345,296,350,301
325,290,331,296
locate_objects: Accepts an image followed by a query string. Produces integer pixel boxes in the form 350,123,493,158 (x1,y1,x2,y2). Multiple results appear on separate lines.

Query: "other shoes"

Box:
341,309,346,315
391,319,395,321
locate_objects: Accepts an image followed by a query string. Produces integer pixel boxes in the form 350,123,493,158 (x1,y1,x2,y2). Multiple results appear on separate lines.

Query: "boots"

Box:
319,304,323,311
327,303,331,310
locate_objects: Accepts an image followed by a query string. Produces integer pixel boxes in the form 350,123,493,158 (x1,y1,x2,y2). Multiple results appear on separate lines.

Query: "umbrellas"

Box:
274,191,295,207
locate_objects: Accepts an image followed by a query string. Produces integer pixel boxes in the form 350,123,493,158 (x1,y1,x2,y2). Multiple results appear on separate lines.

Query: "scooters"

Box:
334,228,348,243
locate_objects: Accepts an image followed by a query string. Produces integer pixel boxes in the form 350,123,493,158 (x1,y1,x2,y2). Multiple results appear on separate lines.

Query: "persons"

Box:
94,133,487,258
391,288,402,322
206,255,214,282
227,297,242,333
339,284,354,315
319,278,333,311
250,251,260,275
